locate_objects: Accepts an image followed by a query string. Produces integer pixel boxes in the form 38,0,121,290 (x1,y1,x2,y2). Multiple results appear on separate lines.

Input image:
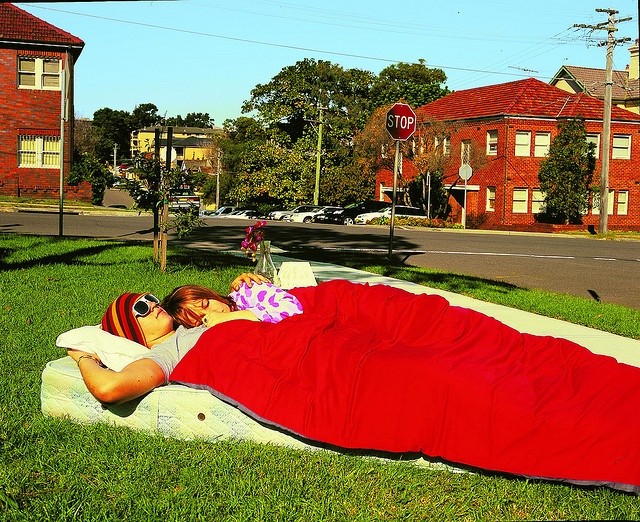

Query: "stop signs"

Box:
385,103,416,141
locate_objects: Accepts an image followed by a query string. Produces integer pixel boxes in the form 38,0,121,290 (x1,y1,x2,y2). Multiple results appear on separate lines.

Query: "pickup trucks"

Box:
209,206,264,216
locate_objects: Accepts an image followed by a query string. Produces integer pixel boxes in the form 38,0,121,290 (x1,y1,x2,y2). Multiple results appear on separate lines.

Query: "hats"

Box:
100,292,149,349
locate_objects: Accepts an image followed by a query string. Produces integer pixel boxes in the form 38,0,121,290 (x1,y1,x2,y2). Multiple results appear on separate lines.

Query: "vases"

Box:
254,240,278,284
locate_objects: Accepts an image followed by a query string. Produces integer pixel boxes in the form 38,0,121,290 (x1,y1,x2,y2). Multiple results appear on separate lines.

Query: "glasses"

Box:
132,293,160,316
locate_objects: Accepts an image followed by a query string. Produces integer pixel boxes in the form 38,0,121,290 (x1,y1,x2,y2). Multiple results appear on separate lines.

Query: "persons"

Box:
65,293,209,407
159,272,299,328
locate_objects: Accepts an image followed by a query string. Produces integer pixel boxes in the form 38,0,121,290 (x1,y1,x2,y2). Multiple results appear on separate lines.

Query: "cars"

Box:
313,207,343,223
290,205,342,223
268,204,302,221
354,204,428,225
324,199,393,225
213,210,264,220
168,187,200,215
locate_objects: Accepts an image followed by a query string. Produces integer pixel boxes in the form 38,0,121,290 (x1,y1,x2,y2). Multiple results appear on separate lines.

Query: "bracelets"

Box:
77,355,101,366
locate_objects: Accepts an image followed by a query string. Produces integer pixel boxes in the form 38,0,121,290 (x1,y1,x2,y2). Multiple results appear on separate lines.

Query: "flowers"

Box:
239,219,274,262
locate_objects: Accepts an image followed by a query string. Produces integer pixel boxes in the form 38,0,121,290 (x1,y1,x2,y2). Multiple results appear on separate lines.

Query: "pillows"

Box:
55,323,150,373
